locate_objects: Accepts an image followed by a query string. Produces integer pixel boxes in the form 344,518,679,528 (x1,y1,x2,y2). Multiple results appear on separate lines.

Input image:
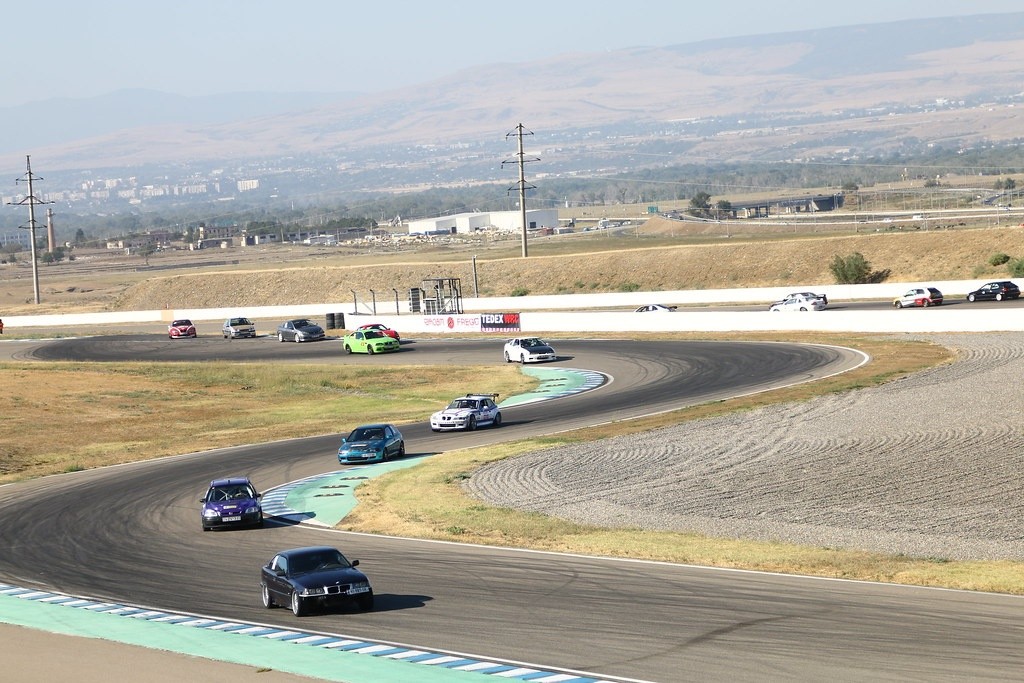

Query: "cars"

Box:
276,318,327,341
168,319,197,339
357,323,400,342
222,318,256,340
966,281,1021,302
664,210,682,220
260,547,374,617
893,288,943,309
583,221,633,231
430,395,502,432
337,425,406,465
343,328,400,354
634,306,674,312
770,291,828,311
504,337,556,364
200,478,263,532
